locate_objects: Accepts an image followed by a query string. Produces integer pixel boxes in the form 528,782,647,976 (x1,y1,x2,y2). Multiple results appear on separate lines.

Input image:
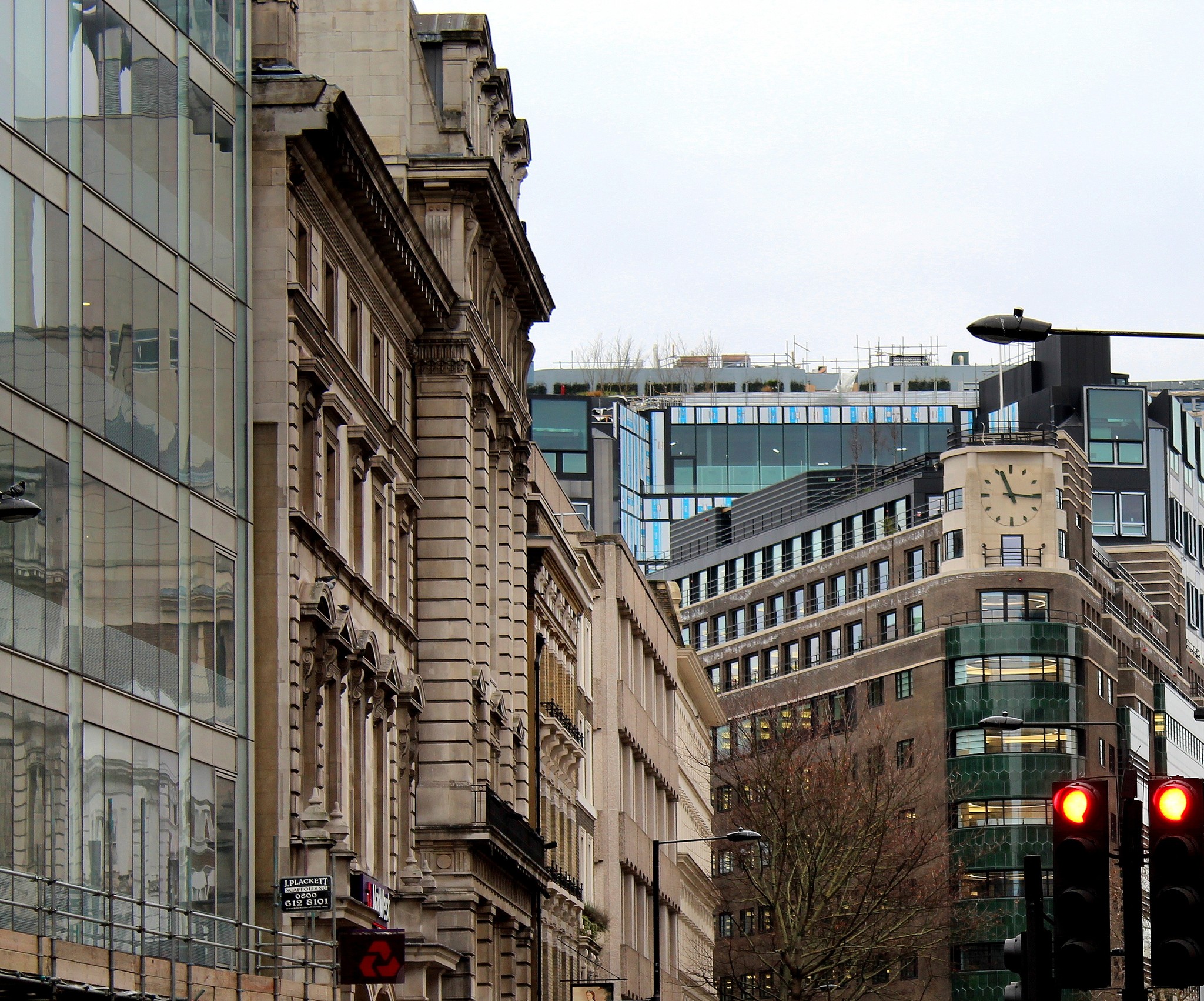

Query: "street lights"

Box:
978,710,1130,774
651,827,762,1001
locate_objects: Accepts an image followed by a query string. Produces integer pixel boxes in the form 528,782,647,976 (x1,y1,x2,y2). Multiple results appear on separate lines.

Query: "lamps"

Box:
1039,544,1045,550
982,543,987,550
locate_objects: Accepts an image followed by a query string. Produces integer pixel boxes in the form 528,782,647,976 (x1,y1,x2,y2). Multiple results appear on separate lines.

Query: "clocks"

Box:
982,464,1042,526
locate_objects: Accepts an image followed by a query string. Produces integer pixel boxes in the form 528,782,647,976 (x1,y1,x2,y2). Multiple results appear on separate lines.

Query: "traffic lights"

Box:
1001,929,1034,1001
1053,778,1111,989
1148,774,1204,990
